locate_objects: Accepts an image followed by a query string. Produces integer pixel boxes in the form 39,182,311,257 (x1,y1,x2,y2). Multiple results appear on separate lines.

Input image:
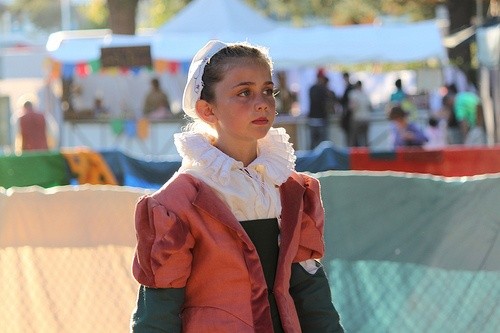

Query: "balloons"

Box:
111,118,148,140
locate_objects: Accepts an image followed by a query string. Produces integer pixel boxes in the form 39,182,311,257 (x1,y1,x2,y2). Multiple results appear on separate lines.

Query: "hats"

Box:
182,39,229,118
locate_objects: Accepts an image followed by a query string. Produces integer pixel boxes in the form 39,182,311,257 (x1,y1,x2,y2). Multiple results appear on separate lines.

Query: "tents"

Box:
43,0,462,161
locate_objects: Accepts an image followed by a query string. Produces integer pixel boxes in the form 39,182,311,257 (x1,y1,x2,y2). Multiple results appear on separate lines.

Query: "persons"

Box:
273,68,480,158
67,72,177,117
129,39,346,333
13,102,53,153
